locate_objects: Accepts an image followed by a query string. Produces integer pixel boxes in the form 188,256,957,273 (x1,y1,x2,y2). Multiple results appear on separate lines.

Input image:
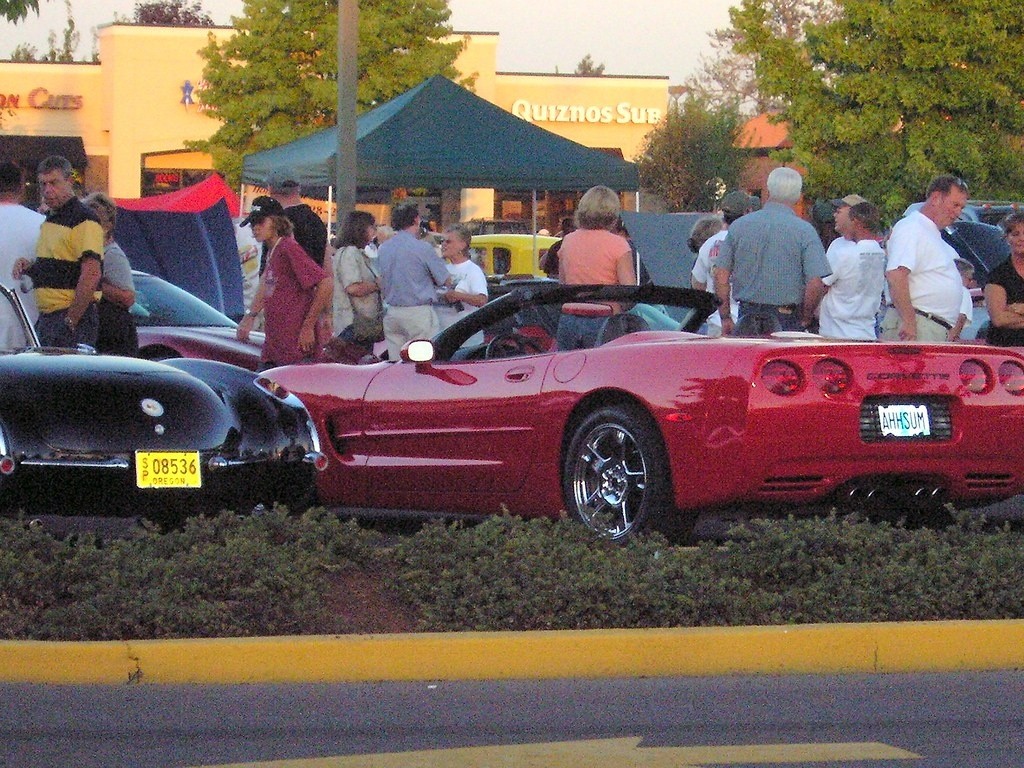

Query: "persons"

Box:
537,215,667,316
0,157,139,358
556,186,638,354
687,168,1024,348
236,181,488,373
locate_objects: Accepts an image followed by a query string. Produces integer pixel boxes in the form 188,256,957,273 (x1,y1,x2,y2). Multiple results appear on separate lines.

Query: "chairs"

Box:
594,313,649,348
733,311,783,337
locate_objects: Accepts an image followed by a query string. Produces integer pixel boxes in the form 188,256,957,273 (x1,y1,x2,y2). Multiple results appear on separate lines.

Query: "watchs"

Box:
245,308,256,317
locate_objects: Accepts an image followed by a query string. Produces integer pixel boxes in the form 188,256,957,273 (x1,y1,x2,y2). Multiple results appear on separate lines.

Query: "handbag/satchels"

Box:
353,312,386,342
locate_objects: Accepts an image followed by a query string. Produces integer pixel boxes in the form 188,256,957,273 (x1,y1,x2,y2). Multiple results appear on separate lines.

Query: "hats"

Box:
829,194,865,207
240,196,286,229
81,192,116,218
722,191,760,215
263,166,302,189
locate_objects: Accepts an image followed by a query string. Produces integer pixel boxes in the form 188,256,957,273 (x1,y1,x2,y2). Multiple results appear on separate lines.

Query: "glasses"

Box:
954,177,969,190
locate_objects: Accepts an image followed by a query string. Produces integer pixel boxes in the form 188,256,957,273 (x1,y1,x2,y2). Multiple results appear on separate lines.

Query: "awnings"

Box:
237,74,641,286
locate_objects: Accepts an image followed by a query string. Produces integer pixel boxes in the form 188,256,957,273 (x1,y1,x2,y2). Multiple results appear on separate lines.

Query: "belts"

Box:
889,303,953,331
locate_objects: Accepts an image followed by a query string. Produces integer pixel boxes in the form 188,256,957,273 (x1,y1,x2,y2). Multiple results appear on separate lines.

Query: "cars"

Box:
0,284,328,534
262,283,1024,546
445,234,562,278
903,200,1024,281
129,269,266,372
488,275,680,332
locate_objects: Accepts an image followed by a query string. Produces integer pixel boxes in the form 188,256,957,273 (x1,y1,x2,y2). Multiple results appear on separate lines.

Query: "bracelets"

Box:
373,281,379,289
63,316,79,326
720,313,731,319
